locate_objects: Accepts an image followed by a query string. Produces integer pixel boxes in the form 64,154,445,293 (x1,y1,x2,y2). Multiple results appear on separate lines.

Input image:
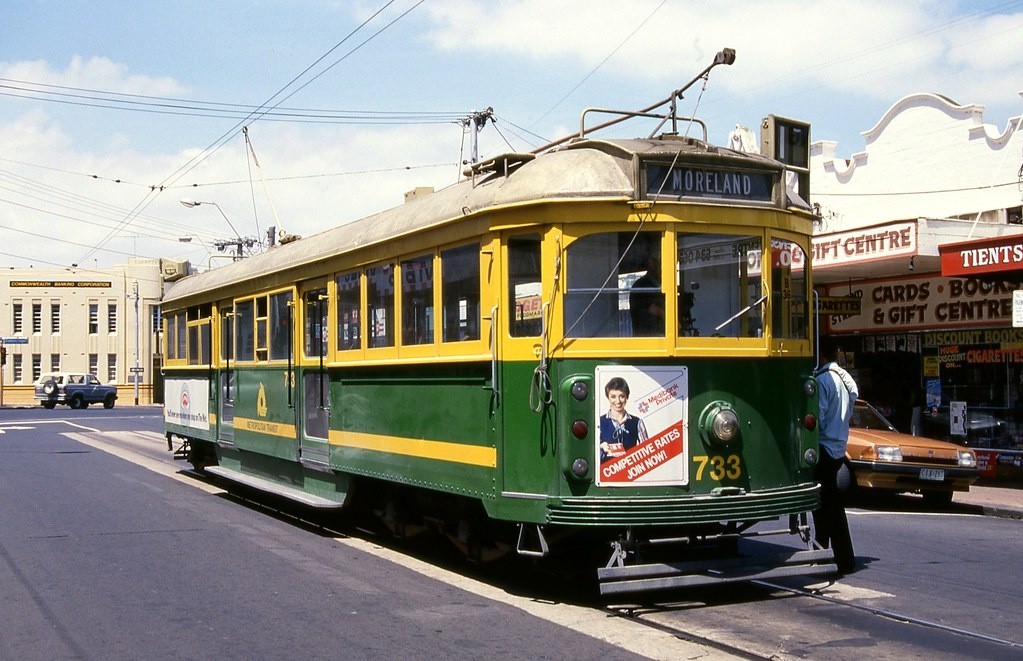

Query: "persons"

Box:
813,334,858,568
629,242,683,336
600,377,648,465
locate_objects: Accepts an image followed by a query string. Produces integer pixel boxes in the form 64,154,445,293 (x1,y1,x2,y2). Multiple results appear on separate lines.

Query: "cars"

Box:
836,398,979,509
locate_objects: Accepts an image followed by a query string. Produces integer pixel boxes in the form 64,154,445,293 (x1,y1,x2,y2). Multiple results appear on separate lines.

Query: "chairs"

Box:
79,376,84,384
57,377,63,384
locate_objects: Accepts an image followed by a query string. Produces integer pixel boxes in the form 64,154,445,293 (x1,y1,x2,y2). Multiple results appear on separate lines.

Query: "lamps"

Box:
908,256,915,271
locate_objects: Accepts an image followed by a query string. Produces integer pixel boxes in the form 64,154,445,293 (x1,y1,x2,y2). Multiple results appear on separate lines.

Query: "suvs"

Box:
33,373,118,409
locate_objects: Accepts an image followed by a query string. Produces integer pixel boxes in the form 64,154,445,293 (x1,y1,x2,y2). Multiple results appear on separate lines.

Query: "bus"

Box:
159,48,839,595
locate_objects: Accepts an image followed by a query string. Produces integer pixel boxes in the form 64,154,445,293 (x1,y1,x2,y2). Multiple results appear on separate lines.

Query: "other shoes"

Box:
840,561,856,572
